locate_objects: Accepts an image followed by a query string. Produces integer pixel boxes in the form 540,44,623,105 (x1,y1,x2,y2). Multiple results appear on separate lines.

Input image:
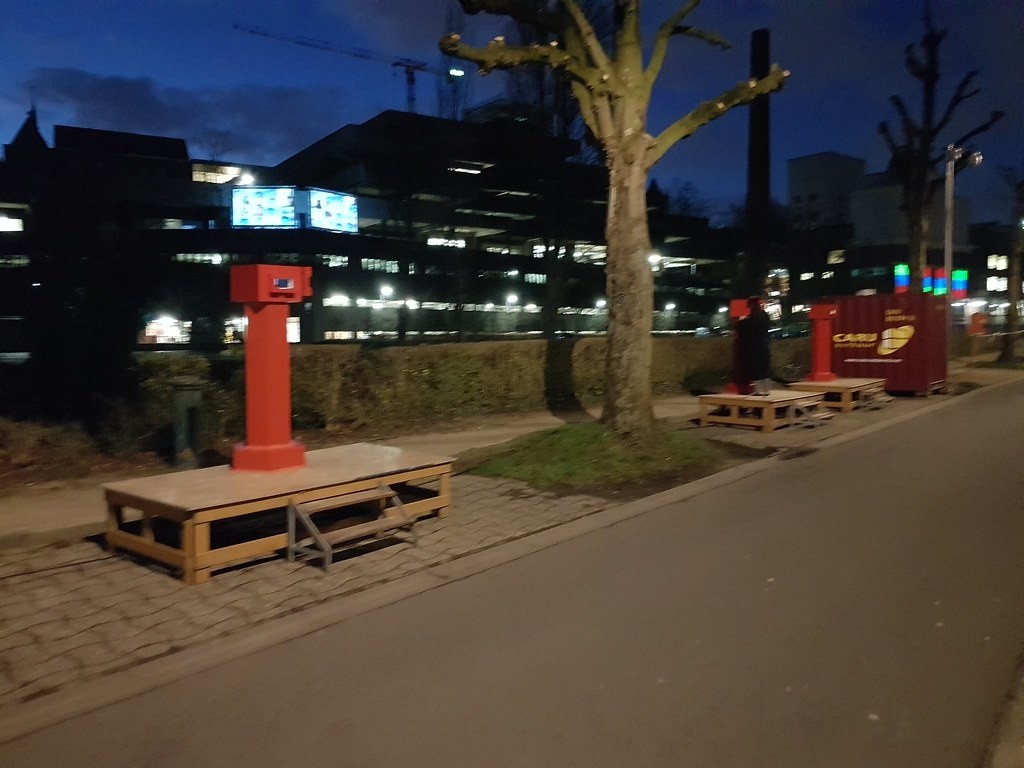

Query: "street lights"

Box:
942,140,984,296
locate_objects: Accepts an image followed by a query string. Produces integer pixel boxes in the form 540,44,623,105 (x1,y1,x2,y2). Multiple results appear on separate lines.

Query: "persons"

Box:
713,278,775,415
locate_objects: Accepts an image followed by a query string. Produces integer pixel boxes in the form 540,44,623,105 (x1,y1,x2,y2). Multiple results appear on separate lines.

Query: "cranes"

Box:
231,19,465,114
